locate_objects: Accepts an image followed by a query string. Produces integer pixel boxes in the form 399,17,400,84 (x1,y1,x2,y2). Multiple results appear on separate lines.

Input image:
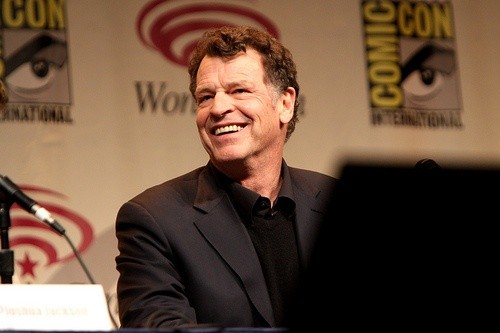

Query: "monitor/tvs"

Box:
291,159,500,333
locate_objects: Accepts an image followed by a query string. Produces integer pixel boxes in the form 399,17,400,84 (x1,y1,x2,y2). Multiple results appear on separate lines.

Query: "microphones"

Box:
0,173,65,236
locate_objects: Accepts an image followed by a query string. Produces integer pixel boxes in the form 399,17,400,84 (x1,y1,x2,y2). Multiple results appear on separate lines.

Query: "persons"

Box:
115,24,341,333
